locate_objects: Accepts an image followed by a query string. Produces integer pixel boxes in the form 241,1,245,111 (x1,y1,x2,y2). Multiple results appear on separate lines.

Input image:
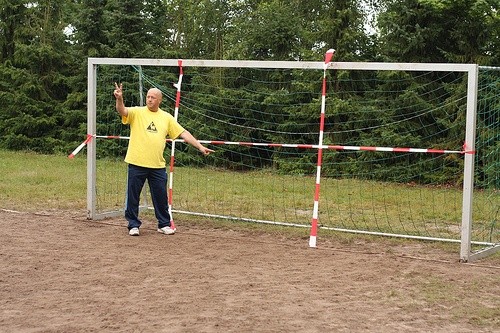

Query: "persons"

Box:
113,82,216,236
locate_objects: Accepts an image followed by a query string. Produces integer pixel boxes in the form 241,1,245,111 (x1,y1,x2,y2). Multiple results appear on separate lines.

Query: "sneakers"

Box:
129,227,140,236
157,225,175,234
